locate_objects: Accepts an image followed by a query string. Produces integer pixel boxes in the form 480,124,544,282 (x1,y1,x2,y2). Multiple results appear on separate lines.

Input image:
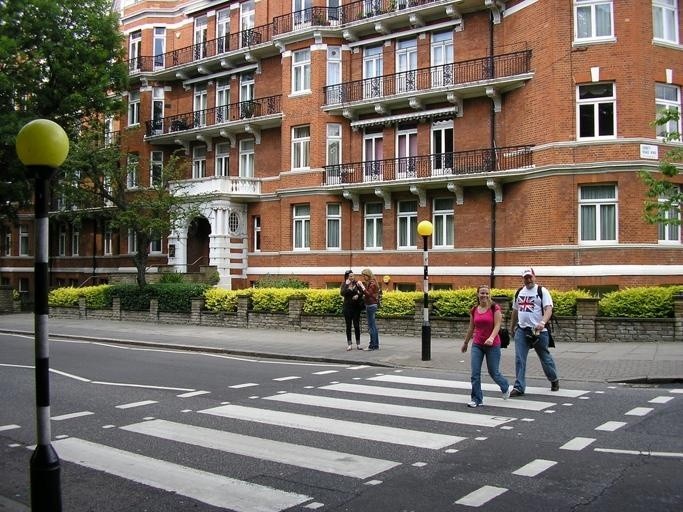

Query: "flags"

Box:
518,296,535,312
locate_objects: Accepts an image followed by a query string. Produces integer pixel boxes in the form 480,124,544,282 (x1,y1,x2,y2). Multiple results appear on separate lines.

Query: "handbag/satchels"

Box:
498,329,510,348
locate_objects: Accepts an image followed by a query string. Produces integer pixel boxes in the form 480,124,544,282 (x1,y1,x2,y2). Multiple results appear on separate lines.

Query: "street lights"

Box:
416,217,434,361
14,116,73,511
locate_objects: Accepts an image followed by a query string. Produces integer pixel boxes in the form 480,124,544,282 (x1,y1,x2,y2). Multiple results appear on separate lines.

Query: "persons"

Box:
357,268,380,351
510,268,560,397
461,284,514,407
340,270,363,351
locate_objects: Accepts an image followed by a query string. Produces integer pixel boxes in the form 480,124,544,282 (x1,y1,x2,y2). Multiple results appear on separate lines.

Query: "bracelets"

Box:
541,321,545,325
464,341,468,344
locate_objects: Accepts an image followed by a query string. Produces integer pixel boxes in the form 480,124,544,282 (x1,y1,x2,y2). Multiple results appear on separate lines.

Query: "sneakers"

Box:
347,345,374,351
503,385,525,400
468,401,482,408
551,380,560,391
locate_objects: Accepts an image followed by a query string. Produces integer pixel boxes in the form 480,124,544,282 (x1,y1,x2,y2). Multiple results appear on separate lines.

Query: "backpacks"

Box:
515,286,555,348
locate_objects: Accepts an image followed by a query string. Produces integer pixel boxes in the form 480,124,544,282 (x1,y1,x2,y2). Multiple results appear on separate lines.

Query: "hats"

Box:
521,268,535,278
